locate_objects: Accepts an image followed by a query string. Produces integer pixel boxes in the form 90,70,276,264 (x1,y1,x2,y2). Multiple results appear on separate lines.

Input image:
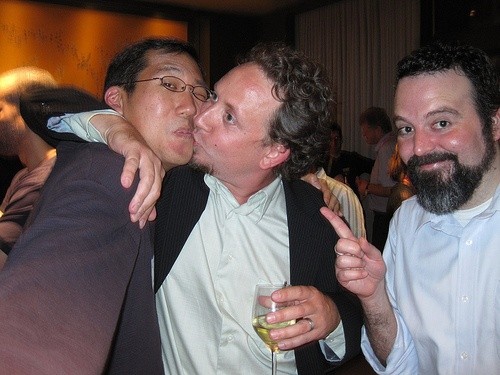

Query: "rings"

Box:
334,245,343,256
304,318,314,330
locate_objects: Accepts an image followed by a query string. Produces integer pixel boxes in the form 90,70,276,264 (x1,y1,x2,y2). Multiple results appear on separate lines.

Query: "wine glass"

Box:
251,284,296,375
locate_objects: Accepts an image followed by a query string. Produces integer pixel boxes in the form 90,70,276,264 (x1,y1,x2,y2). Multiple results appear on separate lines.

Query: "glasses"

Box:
119,75,213,103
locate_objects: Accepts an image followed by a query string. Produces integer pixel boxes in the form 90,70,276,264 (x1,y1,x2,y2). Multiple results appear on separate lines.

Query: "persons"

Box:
320,48,500,375
17,43,365,375
0,39,500,375
